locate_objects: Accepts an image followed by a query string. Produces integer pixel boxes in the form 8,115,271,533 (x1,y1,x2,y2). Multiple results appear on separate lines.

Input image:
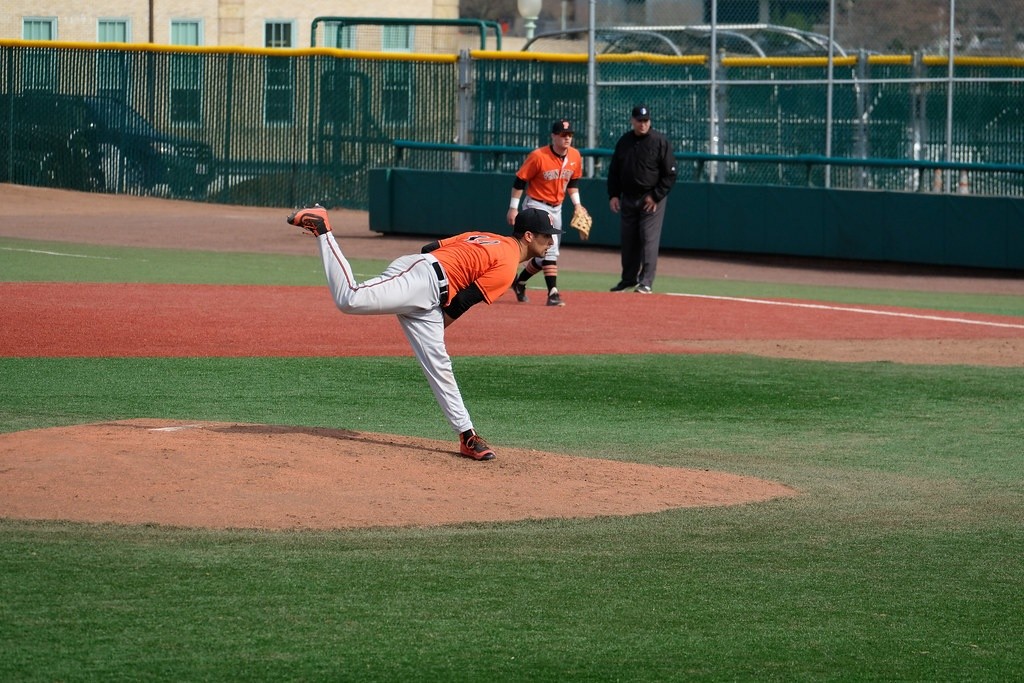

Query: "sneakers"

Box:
288,204,332,239
512,280,529,302
459,429,497,461
634,284,652,294
547,293,566,306
610,281,639,292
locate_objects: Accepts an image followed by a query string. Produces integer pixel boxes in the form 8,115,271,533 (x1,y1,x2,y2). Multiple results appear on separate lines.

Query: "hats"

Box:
515,208,566,235
632,106,650,121
551,119,575,135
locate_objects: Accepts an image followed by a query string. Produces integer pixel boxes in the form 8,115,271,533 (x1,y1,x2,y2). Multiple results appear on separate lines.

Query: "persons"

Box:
286,206,566,459
507,119,586,307
609,105,677,293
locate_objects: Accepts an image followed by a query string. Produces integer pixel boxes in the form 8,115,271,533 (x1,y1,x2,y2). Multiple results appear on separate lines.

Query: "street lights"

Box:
518,0,543,116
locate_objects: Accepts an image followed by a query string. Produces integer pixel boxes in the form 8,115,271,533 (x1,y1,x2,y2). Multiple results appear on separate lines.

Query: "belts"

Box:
432,262,449,307
531,197,560,208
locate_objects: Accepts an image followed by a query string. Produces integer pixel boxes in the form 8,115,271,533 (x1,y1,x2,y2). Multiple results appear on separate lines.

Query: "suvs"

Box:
10,84,219,196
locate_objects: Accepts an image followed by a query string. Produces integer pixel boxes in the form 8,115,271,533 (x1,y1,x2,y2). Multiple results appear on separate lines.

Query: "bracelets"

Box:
570,193,580,206
509,198,519,209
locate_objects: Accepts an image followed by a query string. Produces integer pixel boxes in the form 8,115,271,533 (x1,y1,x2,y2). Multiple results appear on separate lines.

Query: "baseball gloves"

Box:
570,208,594,241
